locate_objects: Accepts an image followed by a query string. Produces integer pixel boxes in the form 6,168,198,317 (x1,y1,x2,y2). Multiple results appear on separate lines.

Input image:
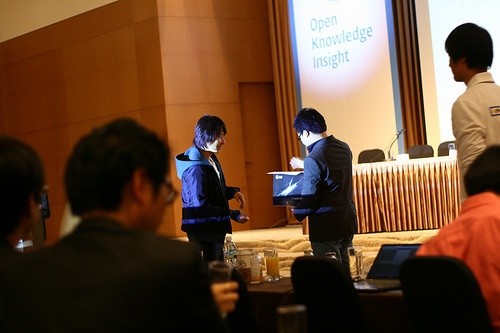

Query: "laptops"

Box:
353,243,423,291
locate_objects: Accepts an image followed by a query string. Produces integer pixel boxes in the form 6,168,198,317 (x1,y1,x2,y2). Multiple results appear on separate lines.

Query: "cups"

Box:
209,261,231,318
234,248,279,284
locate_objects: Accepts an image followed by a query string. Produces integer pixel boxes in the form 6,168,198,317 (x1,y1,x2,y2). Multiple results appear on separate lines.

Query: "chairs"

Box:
408,145,434,158
358,149,385,164
438,141,456,155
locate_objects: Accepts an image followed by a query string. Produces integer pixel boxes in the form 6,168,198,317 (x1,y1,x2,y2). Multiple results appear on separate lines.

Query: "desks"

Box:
242,278,298,305
350,154,458,232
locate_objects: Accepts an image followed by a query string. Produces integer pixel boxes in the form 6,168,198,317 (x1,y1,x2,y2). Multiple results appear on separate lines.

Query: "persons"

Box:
287,108,357,273
416,144,500,333
0,117,239,333
175,115,249,263
445,23,500,199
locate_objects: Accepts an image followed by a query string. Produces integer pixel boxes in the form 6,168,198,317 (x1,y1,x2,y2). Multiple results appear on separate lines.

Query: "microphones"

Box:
396,128,406,135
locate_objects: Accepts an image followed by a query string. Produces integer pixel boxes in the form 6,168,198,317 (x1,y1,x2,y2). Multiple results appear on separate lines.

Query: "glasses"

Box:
160,173,182,206
296,131,304,142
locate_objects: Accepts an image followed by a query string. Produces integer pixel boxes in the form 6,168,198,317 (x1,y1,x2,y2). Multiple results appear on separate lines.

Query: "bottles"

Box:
223,236,238,269
348,247,360,281
325,252,337,260
304,249,314,256
